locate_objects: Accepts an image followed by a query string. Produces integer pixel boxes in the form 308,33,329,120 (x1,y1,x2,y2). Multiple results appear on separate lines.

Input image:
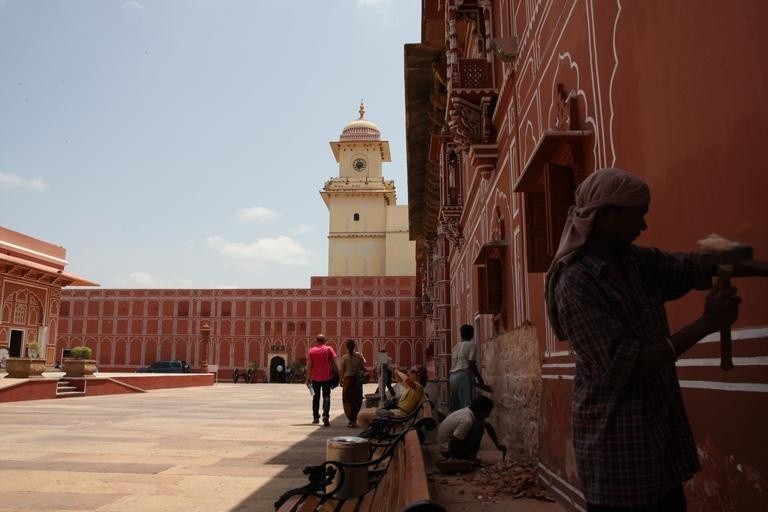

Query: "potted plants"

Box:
24,340,47,378
64,344,97,378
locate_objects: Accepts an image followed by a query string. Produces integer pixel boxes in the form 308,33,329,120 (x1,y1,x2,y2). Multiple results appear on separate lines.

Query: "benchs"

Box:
274,391,448,512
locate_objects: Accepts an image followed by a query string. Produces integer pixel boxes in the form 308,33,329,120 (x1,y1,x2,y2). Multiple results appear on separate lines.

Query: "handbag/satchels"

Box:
357,362,370,384
330,374,339,388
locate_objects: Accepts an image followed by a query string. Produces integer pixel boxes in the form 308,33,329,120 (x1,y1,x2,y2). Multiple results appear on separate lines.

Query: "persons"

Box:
543,168,768,512
306,323,507,467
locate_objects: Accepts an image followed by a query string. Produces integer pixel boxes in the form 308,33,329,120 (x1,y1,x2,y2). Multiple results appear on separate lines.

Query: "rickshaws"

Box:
232,367,252,383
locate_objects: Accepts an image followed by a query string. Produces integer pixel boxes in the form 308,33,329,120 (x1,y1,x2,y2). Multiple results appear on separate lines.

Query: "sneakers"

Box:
312,415,329,426
347,420,357,427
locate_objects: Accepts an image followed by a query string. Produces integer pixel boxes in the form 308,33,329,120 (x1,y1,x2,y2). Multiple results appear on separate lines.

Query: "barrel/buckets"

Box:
326,434,368,499
365,393,381,407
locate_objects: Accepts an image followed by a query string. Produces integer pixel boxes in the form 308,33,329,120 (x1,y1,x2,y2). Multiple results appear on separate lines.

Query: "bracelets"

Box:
664,337,678,362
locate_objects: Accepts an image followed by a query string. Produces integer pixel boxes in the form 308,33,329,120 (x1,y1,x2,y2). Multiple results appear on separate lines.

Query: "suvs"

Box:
133,360,191,372
